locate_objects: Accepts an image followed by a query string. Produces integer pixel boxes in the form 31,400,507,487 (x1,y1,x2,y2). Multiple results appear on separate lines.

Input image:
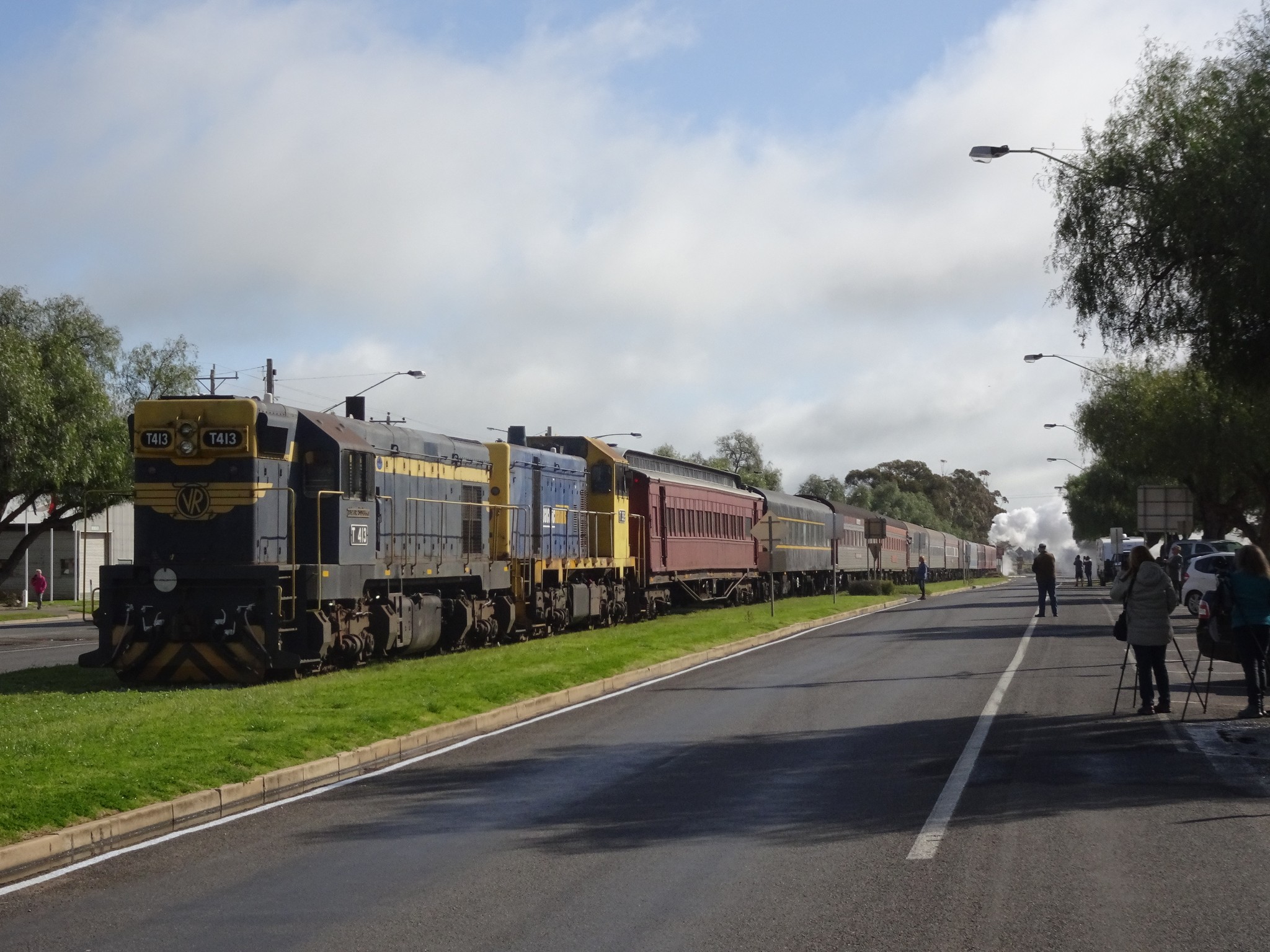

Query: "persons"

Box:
31,569,47,610
1163,545,1184,603
1082,556,1093,587
1073,555,1083,587
1109,545,1177,715
1032,543,1059,618
917,556,928,600
1218,545,1270,719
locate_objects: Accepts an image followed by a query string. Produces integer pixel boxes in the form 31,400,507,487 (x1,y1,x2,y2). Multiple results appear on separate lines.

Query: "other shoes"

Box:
1137,705,1155,715
1053,614,1058,617
917,597,926,600
1238,707,1267,719
1154,697,1171,713
1035,614,1045,617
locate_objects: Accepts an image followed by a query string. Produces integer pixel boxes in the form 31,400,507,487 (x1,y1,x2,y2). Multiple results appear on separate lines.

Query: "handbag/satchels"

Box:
1112,610,1127,641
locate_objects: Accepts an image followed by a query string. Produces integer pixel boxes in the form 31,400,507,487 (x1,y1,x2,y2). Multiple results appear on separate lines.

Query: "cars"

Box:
1167,538,1243,619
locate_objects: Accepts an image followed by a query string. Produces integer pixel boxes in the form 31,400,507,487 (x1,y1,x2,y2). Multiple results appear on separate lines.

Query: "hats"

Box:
1172,545,1181,550
1039,543,1046,548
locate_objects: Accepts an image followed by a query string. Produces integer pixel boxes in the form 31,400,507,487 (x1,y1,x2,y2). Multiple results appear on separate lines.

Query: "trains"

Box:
76,393,1039,689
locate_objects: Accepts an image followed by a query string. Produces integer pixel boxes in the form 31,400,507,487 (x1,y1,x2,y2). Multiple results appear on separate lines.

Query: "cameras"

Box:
1214,556,1231,577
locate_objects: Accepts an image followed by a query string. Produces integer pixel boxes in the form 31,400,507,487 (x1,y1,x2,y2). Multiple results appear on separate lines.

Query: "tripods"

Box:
1111,634,1208,715
1181,577,1270,721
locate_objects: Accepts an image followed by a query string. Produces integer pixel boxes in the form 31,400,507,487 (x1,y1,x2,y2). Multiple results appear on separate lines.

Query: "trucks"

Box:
1096,528,1148,586
